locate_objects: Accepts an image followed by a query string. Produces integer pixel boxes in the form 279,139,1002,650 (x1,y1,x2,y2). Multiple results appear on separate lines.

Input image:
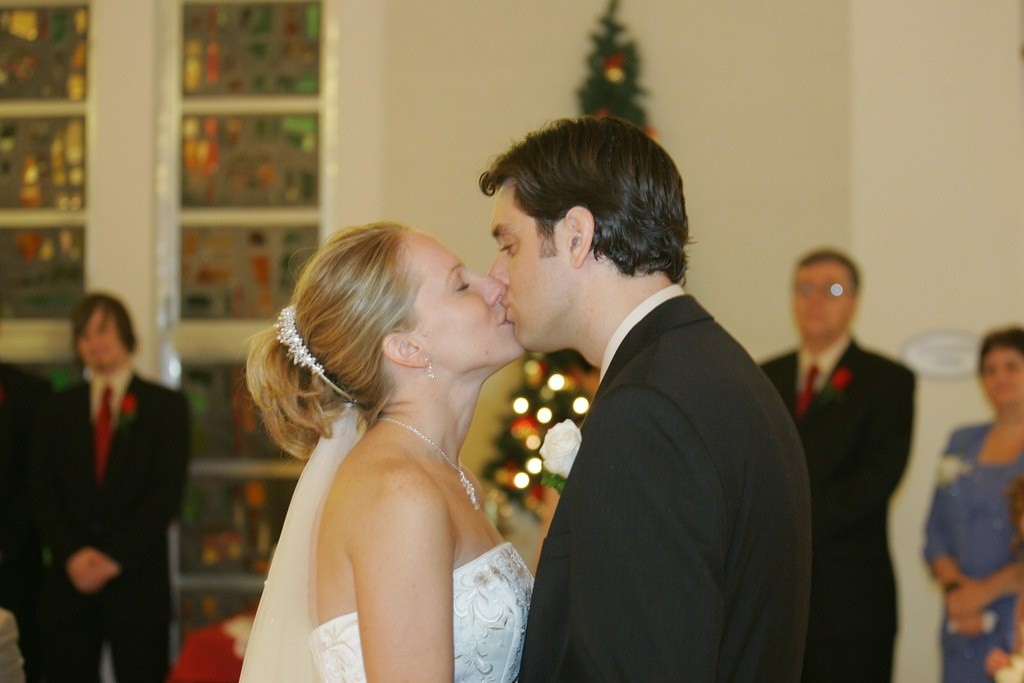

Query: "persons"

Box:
760,252,915,683
479,117,809,683
246,221,532,683
0,362,61,683
23,294,191,683
924,329,1024,683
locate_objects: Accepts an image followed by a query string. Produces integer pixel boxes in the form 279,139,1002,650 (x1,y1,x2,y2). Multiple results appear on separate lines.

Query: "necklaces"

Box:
376,416,480,511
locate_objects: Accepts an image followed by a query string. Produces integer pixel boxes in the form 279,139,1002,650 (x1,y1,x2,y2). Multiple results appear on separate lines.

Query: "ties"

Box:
795,365,820,422
94,386,113,483
